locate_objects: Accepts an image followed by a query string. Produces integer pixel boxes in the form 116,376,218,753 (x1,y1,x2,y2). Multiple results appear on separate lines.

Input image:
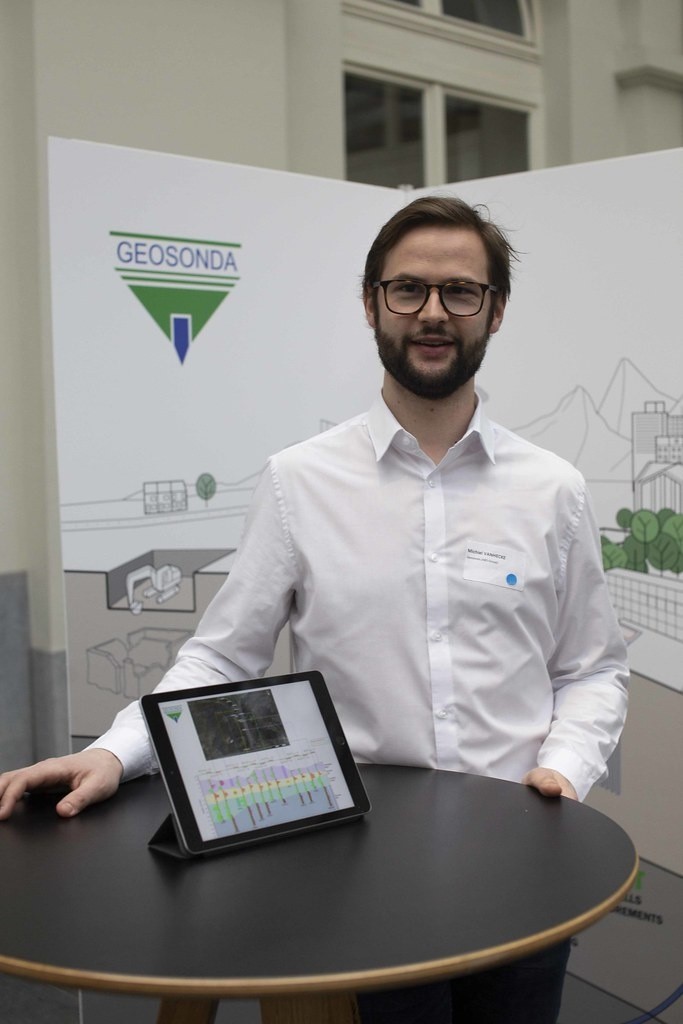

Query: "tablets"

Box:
139,670,372,853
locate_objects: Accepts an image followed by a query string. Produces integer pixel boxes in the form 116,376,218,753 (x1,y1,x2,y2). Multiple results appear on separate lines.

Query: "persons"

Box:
0,195,632,1024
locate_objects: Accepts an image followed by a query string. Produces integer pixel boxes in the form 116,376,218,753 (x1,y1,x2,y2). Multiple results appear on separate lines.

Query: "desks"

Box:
0,760,639,1024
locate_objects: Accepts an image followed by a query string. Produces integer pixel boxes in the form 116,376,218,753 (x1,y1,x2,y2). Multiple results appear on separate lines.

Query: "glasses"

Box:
373,274,501,319
